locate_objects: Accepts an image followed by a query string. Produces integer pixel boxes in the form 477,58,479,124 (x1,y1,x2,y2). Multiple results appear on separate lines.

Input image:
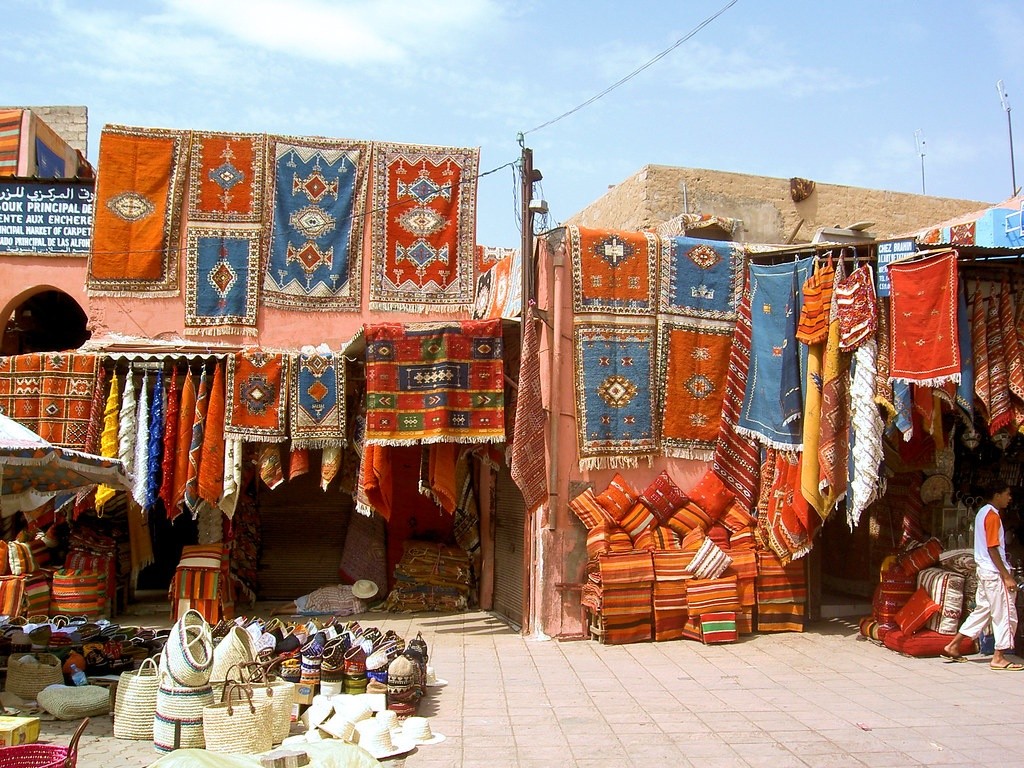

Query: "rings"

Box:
1010,588,1014,591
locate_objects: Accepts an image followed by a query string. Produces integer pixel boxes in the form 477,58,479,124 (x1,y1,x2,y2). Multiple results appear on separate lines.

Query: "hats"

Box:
426,667,448,686
275,694,446,759
352,580,378,598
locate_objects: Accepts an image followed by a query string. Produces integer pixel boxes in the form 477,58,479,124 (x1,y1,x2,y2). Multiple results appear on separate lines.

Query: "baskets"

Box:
0,609,429,768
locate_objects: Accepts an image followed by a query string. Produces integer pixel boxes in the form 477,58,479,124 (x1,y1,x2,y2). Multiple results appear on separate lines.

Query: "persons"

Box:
269,585,381,618
940,479,1024,672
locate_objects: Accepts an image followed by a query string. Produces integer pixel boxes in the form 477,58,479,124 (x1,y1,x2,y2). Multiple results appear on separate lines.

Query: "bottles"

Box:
69,663,88,686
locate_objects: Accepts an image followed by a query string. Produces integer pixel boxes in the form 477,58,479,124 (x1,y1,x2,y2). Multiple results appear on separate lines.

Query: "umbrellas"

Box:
0,412,137,501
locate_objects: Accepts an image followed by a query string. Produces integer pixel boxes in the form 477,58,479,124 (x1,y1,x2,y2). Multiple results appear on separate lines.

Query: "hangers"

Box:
887,244,952,266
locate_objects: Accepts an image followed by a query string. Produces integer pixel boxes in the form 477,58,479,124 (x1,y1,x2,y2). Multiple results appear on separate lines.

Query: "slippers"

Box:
940,655,967,663
991,662,1024,671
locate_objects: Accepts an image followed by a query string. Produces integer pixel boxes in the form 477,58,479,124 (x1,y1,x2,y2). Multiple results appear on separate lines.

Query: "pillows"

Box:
638,469,691,526
585,518,610,560
652,522,682,551
684,575,743,616
894,585,942,636
717,500,757,533
662,499,714,540
682,523,706,551
609,525,633,552
685,536,734,581
705,523,731,550
687,469,736,522
595,472,640,525
900,538,942,576
729,527,757,550
634,526,656,551
0,540,38,576
616,499,659,543
567,487,618,529
176,543,224,568
